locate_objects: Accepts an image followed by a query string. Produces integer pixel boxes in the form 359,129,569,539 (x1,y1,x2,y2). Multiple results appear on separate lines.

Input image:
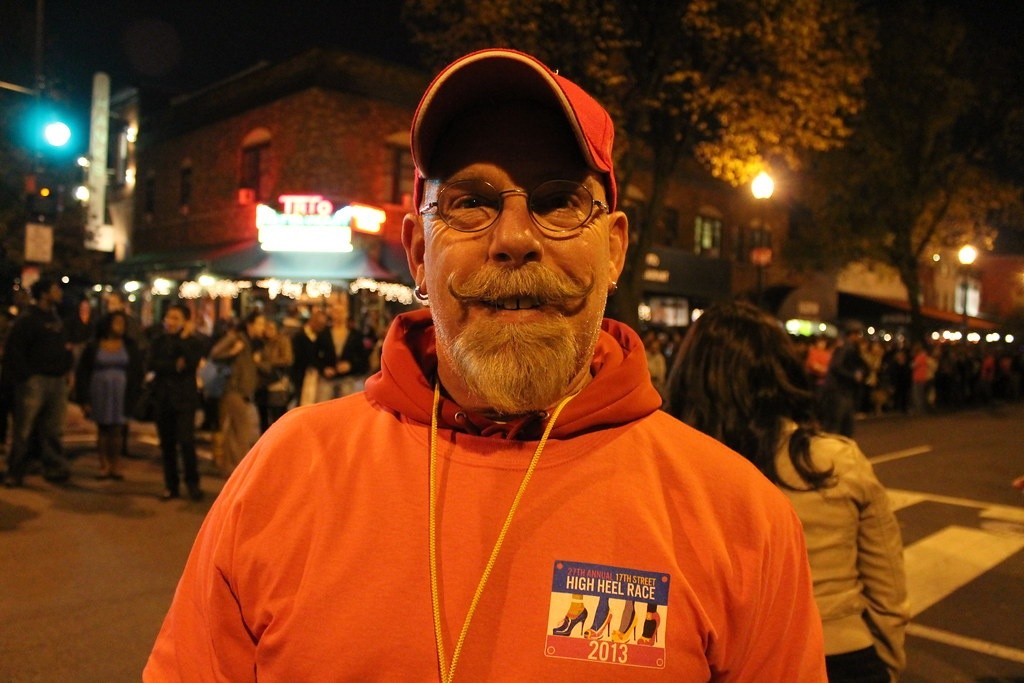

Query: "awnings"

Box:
840,290,1001,332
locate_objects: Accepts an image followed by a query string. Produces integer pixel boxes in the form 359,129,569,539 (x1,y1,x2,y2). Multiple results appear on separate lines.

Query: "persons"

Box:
660,303,907,683
791,319,1024,435
138,304,212,500
1,267,402,487
138,50,825,683
640,329,682,389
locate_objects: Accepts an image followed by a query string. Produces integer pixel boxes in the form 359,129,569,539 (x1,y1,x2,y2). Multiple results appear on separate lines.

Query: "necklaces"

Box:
429,379,586,682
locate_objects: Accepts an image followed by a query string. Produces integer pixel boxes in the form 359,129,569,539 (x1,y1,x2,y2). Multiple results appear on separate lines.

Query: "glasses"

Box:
418,179,607,234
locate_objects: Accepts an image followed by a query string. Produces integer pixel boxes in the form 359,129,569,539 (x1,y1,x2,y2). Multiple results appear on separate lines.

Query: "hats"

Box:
410,48,616,215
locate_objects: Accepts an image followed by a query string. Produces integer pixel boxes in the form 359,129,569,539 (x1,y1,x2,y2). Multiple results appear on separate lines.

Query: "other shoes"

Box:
111,470,124,480
164,489,178,499
189,486,204,500
96,471,110,479
